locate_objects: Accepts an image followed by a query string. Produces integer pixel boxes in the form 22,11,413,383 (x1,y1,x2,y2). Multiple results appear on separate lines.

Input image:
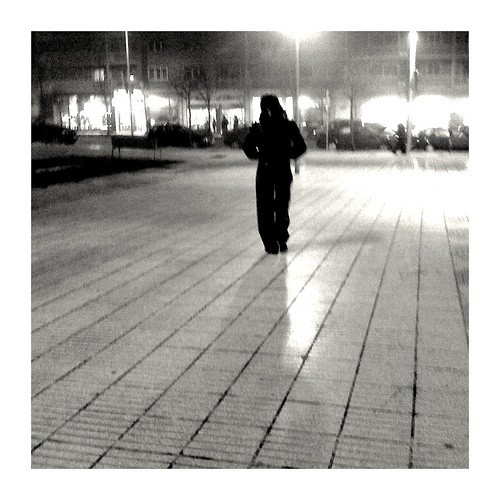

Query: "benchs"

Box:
110,134,162,161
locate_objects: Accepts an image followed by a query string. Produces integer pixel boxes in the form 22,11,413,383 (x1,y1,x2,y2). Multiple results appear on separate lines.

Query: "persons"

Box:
391,124,406,154
234,116,238,129
213,120,216,132
221,116,229,133
242,94,307,254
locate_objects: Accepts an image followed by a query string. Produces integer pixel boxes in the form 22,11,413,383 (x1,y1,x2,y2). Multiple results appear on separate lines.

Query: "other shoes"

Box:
278,238,288,251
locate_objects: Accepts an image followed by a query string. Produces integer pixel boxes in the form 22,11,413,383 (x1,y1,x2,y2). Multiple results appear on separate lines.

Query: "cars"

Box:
144,124,215,148
316,120,379,151
